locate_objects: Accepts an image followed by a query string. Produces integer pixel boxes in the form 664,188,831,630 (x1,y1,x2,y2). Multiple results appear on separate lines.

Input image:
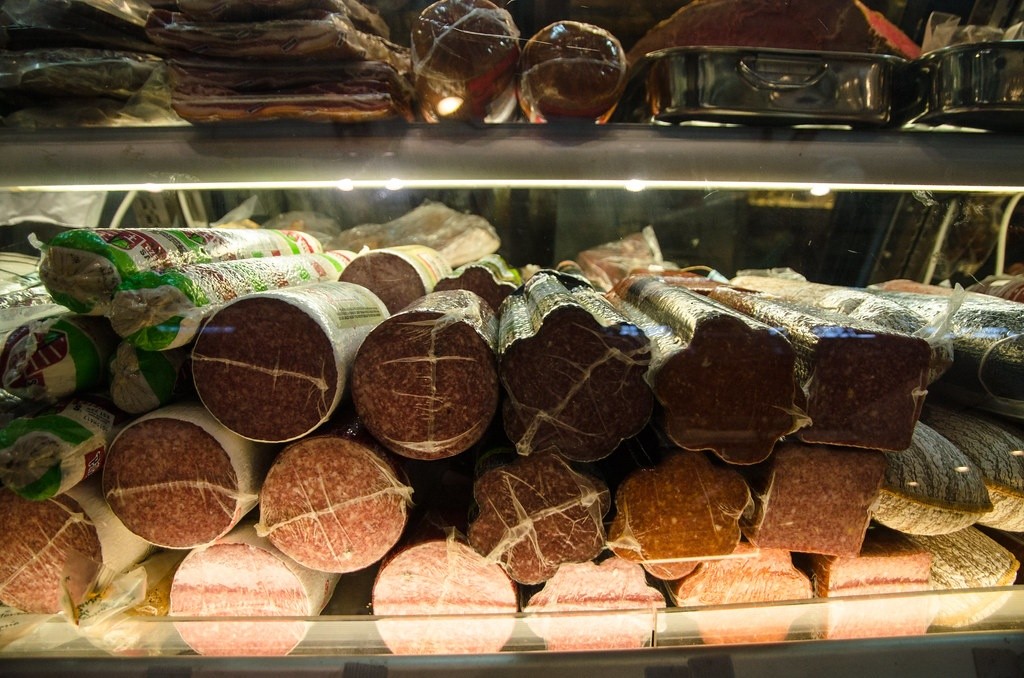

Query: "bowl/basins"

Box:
911,40,1024,120
645,45,911,129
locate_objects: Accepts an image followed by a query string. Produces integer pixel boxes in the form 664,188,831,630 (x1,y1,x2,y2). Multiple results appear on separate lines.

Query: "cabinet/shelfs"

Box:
0,130,1023,677
